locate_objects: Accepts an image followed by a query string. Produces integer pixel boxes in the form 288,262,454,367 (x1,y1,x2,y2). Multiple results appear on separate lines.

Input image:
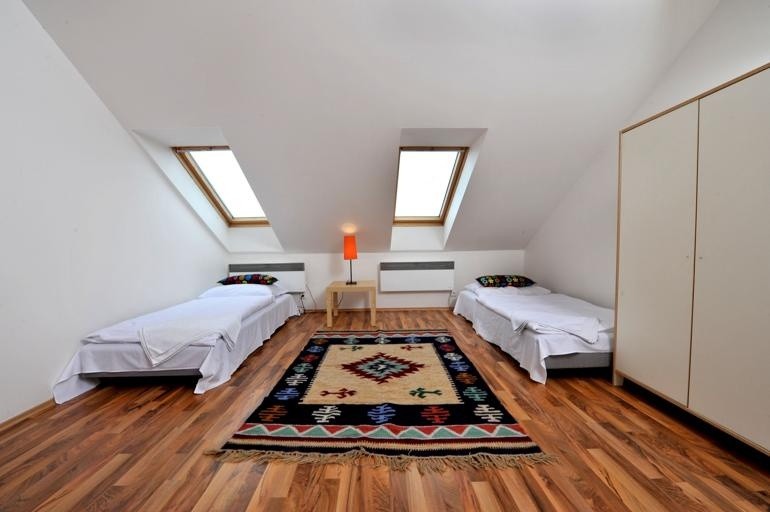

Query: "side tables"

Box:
326,281,377,327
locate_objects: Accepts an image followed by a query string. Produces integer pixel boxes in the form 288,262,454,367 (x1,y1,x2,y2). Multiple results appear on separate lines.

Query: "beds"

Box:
79,294,300,377
453,290,613,378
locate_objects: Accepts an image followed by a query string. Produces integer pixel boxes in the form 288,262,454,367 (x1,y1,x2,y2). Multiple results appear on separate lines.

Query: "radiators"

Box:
228,264,305,293
380,261,454,292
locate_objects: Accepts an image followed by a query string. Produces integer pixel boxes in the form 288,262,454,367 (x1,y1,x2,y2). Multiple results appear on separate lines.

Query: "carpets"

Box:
202,330,560,477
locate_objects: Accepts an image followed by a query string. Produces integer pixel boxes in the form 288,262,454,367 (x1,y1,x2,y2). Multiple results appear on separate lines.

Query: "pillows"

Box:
465,282,551,298
199,284,288,298
476,274,536,287
217,274,278,285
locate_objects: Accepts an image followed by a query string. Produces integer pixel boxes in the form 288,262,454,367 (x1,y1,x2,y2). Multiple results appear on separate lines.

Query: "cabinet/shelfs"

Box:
613,63,770,458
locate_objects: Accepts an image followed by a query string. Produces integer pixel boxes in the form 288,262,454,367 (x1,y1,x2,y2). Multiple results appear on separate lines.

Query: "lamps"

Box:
343,236,358,284
